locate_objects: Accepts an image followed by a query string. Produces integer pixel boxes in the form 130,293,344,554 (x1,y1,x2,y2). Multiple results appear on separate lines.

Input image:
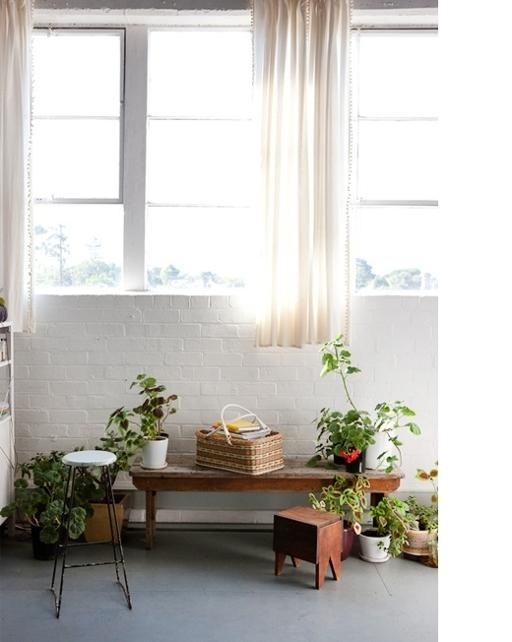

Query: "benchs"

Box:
130,455,409,550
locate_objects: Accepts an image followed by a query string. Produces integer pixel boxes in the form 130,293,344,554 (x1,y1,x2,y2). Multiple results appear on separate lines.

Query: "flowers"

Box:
320,333,421,473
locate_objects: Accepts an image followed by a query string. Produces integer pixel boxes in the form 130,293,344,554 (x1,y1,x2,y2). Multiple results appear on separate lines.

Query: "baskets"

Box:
193,403,285,477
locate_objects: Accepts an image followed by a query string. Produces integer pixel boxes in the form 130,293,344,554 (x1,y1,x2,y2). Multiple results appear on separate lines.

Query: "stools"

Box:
273,506,344,588
50,450,133,619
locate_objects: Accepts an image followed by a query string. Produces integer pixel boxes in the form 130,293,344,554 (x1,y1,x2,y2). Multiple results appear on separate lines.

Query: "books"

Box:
209,418,270,440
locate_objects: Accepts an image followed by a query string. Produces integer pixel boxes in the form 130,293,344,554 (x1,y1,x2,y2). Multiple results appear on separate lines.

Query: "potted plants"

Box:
305,407,375,470
107,376,179,468
1,445,130,560
309,469,438,567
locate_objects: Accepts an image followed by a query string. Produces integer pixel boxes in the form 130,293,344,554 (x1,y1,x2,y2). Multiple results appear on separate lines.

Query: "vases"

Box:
345,447,362,472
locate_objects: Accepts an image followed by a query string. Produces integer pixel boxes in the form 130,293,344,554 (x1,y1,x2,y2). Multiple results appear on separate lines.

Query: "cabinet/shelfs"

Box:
1,321,17,525
